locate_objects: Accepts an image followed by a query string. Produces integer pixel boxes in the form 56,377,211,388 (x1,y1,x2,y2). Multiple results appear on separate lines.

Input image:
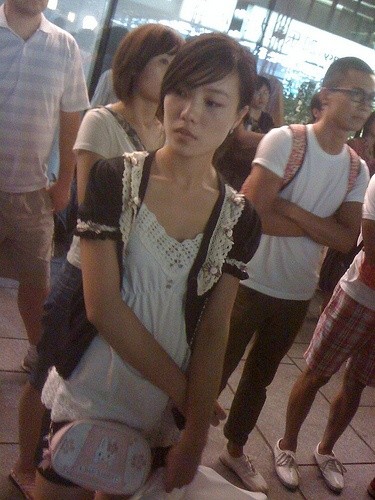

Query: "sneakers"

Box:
312,441,347,492
273,437,302,491
219,445,269,494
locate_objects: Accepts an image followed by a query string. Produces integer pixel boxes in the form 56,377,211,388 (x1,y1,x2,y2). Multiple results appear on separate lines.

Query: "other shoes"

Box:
21,347,40,373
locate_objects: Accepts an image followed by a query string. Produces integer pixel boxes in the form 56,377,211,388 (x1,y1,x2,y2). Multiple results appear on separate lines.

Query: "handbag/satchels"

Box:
49,418,152,496
127,463,269,500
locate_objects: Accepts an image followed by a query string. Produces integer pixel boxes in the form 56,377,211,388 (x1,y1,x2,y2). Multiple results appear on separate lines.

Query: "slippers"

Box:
9,465,36,500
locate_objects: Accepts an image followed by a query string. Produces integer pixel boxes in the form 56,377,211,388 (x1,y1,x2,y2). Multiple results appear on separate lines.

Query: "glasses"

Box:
327,86,375,113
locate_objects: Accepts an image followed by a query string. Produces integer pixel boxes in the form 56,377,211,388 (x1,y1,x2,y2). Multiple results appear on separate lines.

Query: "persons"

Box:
0,0,91,374
89,70,119,107
310,92,323,123
217,55,375,494
9,23,187,500
34,31,264,500
317,111,375,294
241,74,275,134
271,172,375,493
